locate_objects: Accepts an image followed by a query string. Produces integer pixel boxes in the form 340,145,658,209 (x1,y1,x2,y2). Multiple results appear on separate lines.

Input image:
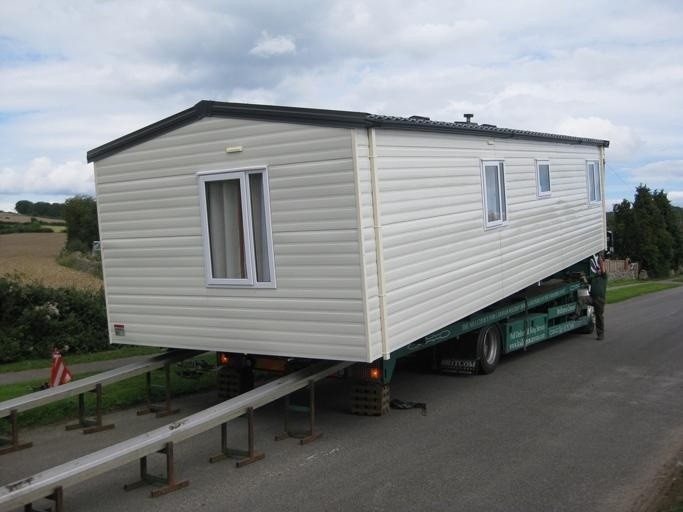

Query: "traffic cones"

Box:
48,350,74,390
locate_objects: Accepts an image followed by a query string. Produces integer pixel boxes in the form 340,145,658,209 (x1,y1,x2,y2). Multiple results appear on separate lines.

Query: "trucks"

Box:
213,225,615,387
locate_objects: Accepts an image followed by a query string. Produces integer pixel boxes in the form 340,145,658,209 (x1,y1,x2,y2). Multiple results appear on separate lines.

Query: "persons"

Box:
569,255,607,340
